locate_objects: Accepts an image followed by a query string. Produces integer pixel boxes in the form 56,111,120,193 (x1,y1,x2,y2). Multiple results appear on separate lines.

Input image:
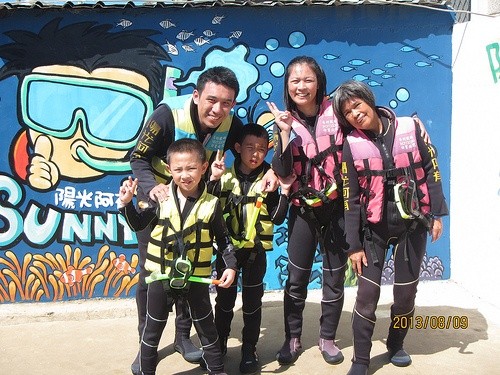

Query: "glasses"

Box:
393,179,424,221
169,240,194,290
226,193,246,241
302,160,337,206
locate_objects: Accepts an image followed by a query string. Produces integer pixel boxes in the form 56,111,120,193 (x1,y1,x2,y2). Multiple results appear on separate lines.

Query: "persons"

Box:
266,55,431,365
131,66,280,375
331,80,449,375
119,137,239,375
207,122,297,375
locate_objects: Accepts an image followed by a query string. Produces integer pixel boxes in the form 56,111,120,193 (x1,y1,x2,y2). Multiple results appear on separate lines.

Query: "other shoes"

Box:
347,359,369,375
386,343,414,367
319,336,343,365
173,337,204,362
199,344,227,367
131,354,142,375
240,346,262,374
275,337,301,365
